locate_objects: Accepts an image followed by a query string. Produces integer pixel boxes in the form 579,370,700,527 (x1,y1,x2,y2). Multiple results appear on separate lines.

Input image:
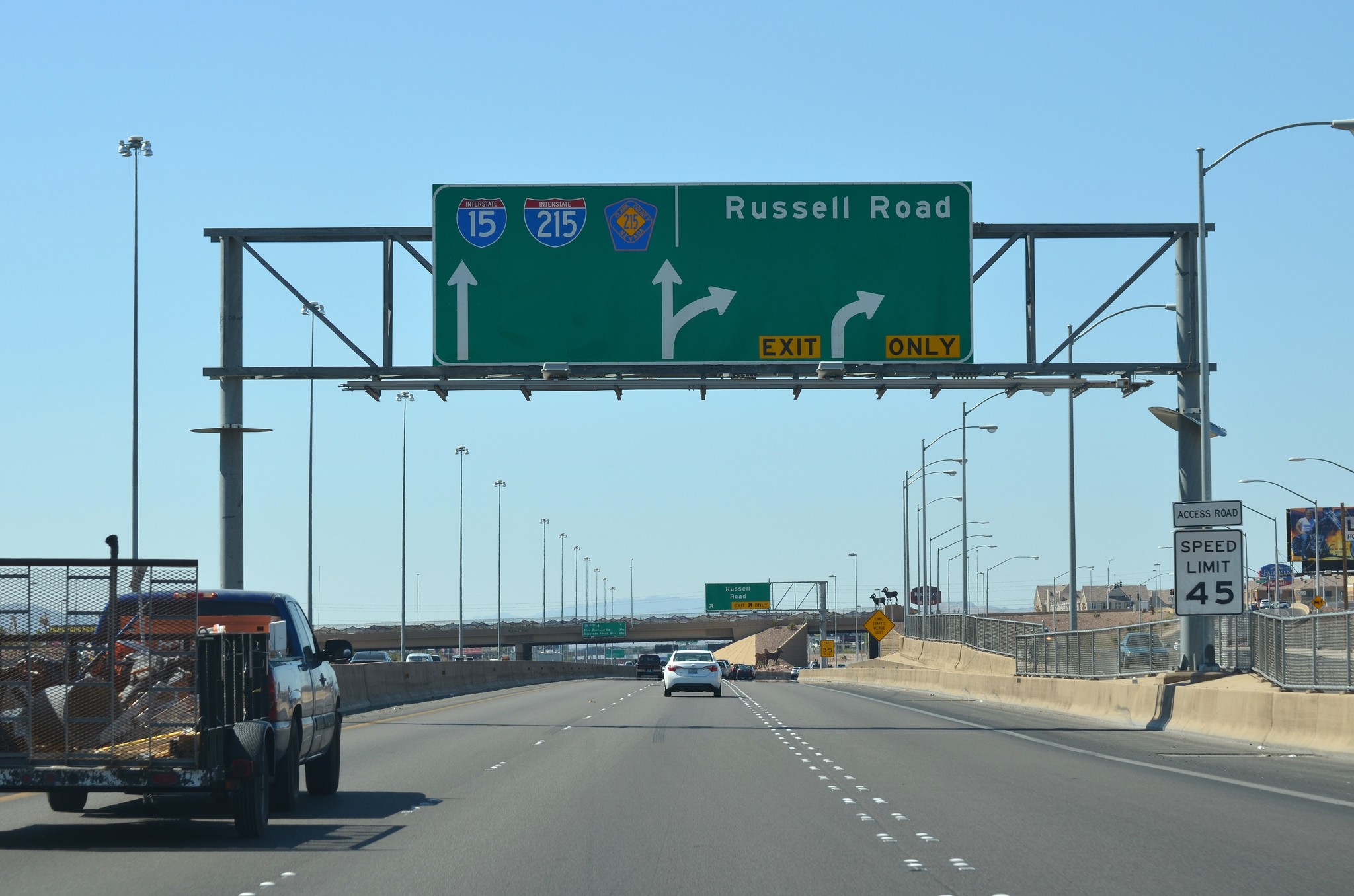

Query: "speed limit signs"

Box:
1174,529,1243,615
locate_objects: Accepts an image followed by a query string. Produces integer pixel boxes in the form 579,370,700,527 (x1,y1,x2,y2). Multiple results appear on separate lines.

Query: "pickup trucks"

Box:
0,589,353,839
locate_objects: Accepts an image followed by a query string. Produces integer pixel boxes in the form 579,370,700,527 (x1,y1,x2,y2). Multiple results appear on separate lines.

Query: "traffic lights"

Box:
1267,586,1269,593
1170,589,1174,595
1114,582,1122,589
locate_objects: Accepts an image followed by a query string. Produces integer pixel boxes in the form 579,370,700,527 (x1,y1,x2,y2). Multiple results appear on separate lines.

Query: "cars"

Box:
659,650,725,698
791,661,845,681
1248,603,1259,610
617,649,756,681
335,650,474,664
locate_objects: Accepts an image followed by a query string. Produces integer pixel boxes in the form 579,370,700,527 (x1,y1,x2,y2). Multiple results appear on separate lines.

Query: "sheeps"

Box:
882,587,899,605
755,648,768,668
870,593,886,609
766,648,783,666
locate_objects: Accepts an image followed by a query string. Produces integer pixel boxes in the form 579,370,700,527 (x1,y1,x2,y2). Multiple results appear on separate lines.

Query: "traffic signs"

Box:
432,182,974,367
705,582,771,613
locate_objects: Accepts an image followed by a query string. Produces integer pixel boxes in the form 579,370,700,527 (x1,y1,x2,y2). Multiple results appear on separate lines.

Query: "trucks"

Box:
537,652,563,662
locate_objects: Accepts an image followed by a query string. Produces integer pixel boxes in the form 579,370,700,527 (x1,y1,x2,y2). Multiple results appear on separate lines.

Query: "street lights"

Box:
301,300,326,628
902,305,1174,645
1238,479,1318,610
119,135,154,595
1250,578,1285,611
829,552,894,668
416,573,420,625
1194,117,1354,672
396,391,615,666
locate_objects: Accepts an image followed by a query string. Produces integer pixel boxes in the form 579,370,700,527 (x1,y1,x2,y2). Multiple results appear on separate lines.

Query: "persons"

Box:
1295,510,1333,560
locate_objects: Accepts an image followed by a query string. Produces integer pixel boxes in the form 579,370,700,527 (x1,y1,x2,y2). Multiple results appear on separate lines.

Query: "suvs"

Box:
1260,599,1271,609
1270,601,1290,609
1120,633,1170,670
